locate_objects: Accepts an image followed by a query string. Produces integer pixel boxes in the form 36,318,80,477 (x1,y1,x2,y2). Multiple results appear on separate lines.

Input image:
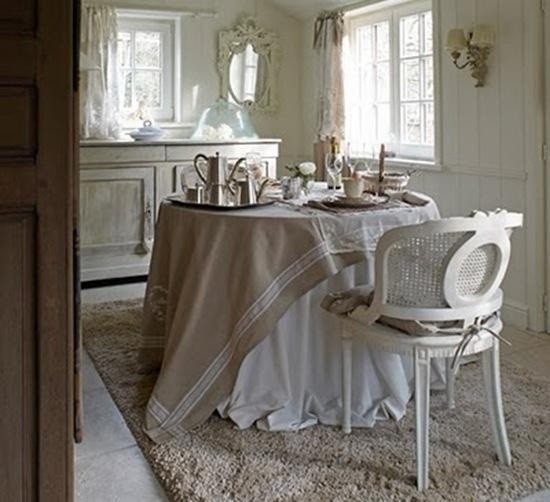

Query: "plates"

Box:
124,132,167,141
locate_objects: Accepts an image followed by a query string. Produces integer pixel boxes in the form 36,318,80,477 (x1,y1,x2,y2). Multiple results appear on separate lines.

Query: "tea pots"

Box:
182,151,269,206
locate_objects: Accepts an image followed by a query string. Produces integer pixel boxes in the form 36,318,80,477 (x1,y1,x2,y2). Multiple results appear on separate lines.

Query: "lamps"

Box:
446,27,497,88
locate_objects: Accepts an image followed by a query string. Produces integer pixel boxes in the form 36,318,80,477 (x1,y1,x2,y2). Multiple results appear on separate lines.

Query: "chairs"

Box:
323,206,524,492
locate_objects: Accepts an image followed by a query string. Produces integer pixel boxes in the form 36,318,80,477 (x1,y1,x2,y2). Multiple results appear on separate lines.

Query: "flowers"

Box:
287,158,317,185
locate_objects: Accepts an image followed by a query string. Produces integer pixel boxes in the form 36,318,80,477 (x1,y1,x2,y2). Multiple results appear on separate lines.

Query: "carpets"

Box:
77,298,547,500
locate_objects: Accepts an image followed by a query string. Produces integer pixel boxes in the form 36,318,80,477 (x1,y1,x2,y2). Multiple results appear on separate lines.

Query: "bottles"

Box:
327,135,342,191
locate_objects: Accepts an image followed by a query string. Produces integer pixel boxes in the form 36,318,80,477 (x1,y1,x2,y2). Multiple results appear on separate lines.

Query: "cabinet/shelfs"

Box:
77,147,160,286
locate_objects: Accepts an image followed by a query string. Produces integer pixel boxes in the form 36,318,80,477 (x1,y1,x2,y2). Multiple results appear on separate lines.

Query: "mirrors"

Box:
216,16,285,115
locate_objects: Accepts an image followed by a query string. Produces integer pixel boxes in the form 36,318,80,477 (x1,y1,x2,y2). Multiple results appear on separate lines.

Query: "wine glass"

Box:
324,151,341,196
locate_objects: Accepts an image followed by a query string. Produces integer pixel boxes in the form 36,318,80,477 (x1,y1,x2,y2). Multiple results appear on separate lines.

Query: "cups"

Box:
343,179,367,199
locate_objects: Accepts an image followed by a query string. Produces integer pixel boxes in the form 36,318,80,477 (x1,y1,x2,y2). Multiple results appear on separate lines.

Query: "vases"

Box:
284,176,304,198
